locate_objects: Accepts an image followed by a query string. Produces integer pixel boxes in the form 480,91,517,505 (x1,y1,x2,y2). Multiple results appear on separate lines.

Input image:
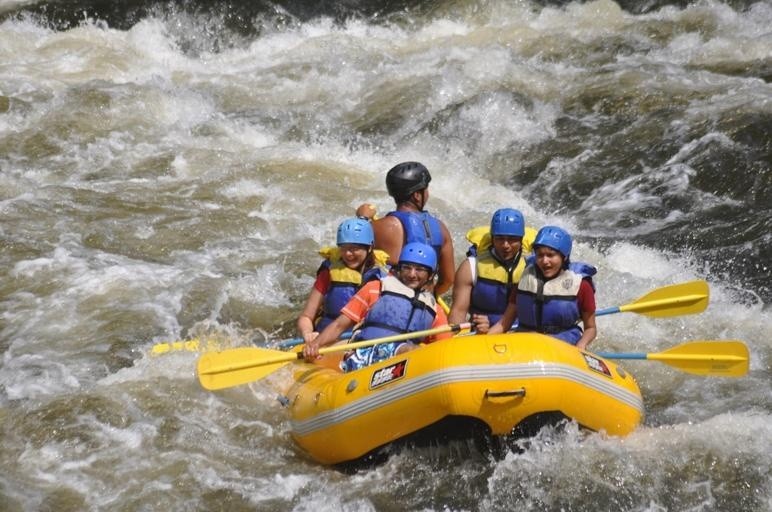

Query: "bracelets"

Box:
359,215,369,220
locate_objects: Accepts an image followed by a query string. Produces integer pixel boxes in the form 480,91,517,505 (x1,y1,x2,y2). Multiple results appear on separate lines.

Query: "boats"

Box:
277,326,647,474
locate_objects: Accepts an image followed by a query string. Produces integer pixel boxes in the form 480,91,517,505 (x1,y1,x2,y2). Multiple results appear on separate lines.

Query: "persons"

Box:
303,241,453,372
356,161,455,295
446,208,527,333
486,226,596,349
296,217,387,346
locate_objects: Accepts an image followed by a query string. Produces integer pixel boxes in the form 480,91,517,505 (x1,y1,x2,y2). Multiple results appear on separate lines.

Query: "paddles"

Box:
595,340,750,377
151,332,354,353
197,321,476,391
471,281,709,332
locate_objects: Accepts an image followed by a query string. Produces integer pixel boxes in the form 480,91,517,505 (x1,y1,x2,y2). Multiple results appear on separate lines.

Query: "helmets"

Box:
386,162,431,195
398,242,438,270
336,216,375,246
534,224,570,260
490,210,526,237
